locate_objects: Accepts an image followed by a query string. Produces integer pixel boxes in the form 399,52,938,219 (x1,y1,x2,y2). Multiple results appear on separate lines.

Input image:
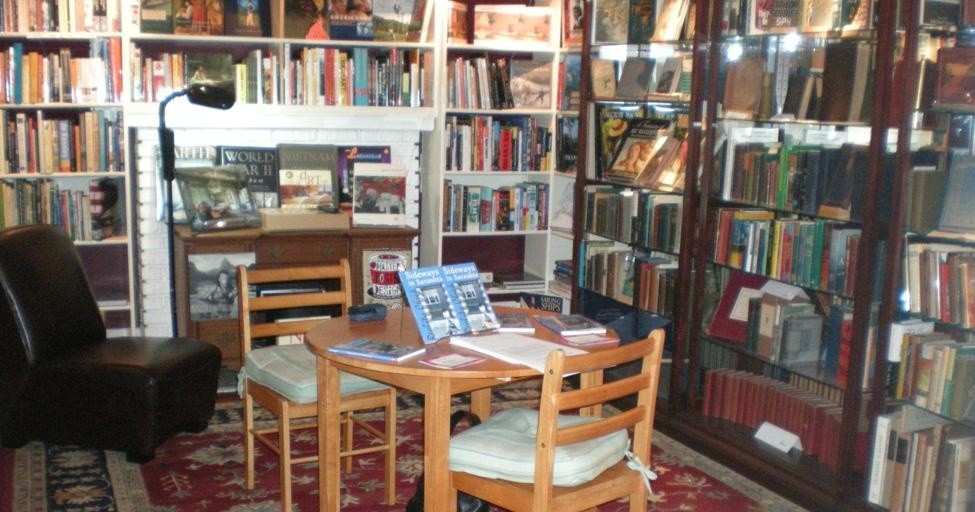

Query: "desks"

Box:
306,300,622,512
174,221,422,388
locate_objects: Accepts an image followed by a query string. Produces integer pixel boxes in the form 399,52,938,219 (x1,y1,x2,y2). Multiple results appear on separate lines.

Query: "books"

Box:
130,0,431,108
560,1,692,346
865,1,975,511
702,1,874,477
331,262,608,364
155,143,411,319
1,0,125,242
444,2,554,232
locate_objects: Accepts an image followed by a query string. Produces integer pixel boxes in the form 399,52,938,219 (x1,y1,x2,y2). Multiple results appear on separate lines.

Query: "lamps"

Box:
159,78,238,336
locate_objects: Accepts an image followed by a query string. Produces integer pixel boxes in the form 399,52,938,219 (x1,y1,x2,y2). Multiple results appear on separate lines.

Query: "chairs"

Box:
451,326,666,512
0,225,222,465
237,258,400,512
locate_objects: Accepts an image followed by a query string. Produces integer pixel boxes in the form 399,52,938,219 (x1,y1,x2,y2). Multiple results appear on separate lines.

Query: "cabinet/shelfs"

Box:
0,0,136,338
679,0,891,511
857,0,975,511
419,1,561,295
569,0,704,432
125,0,441,111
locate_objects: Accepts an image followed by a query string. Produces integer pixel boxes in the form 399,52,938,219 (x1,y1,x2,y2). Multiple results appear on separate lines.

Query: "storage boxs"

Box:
258,205,350,231
